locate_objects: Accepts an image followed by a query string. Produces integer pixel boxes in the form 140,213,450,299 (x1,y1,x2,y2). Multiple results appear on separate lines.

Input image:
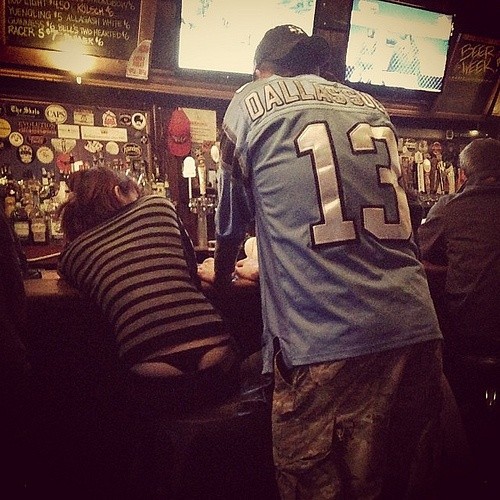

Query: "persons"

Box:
57,166,253,414
214,24,464,500
416,137,500,339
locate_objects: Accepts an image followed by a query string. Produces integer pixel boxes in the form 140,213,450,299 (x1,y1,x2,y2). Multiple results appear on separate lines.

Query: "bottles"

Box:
3,158,171,245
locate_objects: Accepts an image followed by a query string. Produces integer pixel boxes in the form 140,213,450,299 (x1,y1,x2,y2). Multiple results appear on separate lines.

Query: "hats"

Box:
253,25,331,69
168,110,192,156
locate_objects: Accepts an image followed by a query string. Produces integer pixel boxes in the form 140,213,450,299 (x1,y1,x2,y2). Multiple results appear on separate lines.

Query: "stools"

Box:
132,376,270,499
451,349,499,468
